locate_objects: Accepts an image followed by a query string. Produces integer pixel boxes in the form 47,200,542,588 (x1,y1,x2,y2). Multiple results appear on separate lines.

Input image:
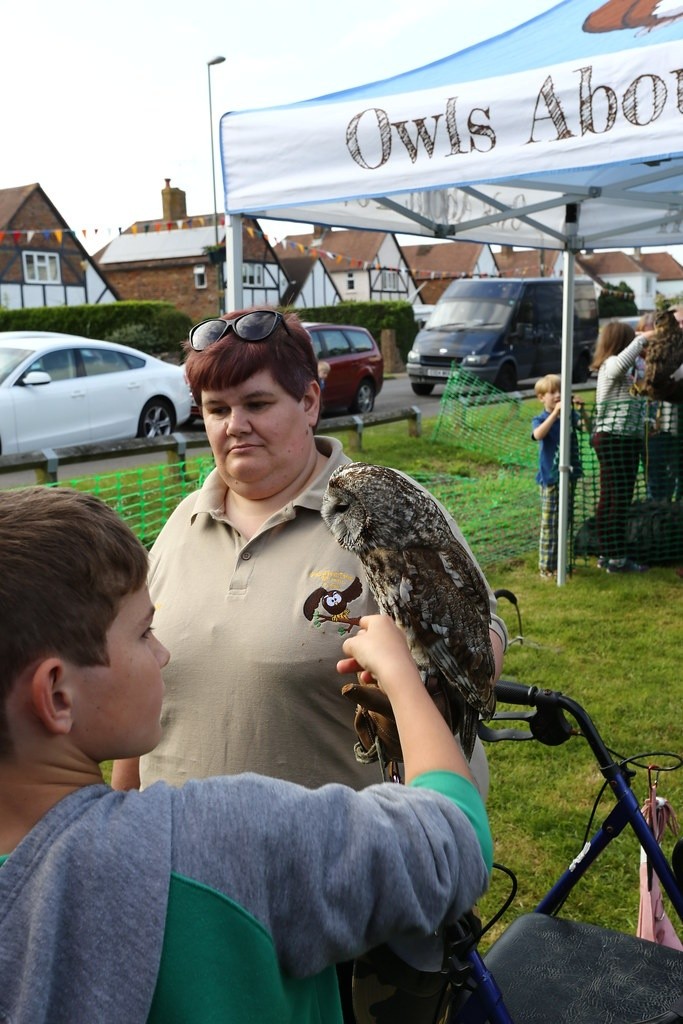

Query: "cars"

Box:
0,330,191,458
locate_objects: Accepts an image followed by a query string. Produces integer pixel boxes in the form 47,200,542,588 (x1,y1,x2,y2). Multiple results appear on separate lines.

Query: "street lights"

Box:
206,54,226,317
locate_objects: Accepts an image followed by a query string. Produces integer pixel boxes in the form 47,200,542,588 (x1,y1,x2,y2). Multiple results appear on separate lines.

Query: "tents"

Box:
216,0,682,591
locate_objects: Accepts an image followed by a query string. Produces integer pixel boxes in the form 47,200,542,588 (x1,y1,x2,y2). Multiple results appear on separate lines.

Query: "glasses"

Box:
189,308,316,380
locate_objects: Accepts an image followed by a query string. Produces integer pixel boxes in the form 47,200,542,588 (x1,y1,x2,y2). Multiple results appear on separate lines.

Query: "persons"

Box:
588,302,683,574
531,374,589,578
109,306,506,804
0,486,494,1024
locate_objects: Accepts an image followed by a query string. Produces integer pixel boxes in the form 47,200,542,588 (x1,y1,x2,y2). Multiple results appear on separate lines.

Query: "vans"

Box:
405,276,596,397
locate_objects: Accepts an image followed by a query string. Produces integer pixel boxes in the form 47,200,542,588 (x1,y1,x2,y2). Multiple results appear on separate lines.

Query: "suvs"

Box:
179,320,385,428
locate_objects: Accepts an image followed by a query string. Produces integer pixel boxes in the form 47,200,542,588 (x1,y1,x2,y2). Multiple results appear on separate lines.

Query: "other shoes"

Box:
597,556,624,573
540,570,557,578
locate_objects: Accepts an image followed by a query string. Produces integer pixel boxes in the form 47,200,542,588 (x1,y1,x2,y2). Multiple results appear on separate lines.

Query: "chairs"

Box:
336,678,683,1024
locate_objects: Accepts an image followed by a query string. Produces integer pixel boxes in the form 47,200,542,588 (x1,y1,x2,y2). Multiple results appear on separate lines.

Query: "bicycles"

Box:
450,681,683,1023
